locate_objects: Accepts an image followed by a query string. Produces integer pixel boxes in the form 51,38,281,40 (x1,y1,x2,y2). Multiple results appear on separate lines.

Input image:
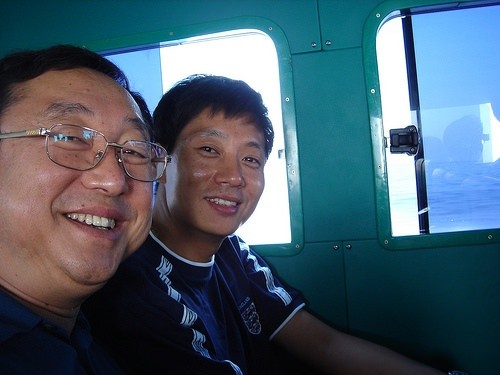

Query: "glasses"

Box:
0,122,173,184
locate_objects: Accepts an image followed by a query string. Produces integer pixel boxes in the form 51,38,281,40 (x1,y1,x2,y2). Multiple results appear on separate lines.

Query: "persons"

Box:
89,75,447,375
1,45,169,374
